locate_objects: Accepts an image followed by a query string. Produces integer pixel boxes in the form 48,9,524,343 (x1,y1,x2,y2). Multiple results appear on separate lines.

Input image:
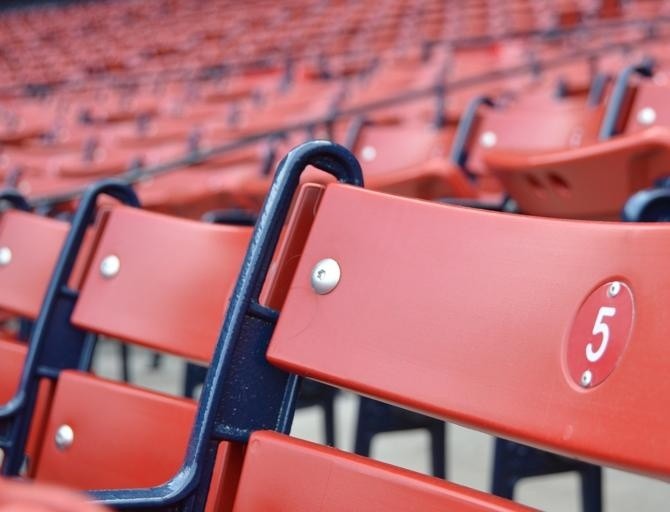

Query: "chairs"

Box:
1,0,670,511
